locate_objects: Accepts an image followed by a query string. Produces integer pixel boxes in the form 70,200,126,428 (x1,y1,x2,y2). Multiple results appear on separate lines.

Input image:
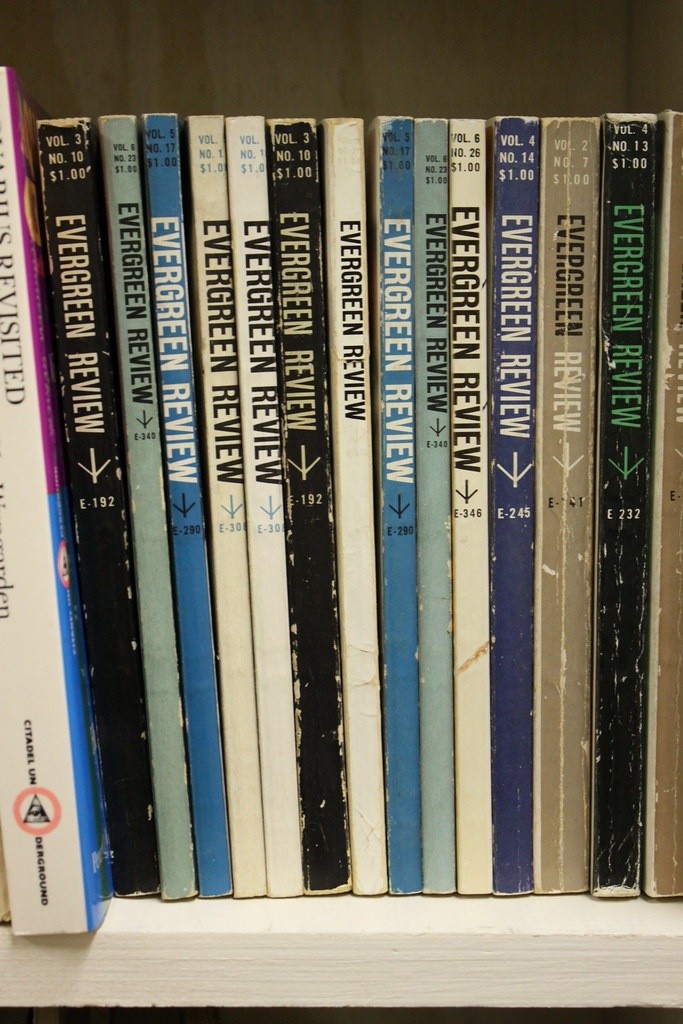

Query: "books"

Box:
1,58,683,940
